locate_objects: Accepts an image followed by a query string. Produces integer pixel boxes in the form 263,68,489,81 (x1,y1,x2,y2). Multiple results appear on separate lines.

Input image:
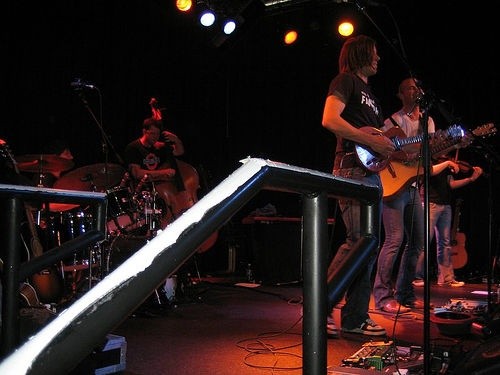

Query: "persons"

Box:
301,36,396,336
374,77,434,312
412,160,482,286
125,119,185,180
44,142,74,188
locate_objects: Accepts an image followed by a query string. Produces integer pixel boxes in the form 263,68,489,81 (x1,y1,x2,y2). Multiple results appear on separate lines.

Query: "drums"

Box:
32,186,167,305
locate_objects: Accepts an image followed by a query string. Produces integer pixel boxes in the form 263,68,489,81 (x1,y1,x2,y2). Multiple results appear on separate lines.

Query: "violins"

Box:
432,155,478,172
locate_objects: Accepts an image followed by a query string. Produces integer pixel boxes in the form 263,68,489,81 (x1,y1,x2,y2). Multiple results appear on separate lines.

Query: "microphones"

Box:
70,82,96,90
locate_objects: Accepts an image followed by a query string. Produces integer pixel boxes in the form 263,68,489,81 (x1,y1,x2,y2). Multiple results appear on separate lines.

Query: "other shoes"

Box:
402,299,434,309
381,301,411,313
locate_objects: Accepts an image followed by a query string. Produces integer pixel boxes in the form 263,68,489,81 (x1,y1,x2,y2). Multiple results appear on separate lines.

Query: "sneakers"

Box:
438,279,465,286
324,313,338,334
342,319,387,335
412,275,425,286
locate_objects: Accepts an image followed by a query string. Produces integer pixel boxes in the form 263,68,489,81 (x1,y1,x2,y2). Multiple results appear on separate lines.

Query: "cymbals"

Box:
42,163,125,212
10,153,75,173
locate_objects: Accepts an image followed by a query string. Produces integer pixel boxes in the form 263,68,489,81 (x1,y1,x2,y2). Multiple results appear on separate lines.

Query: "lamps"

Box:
216,8,237,37
195,1,216,27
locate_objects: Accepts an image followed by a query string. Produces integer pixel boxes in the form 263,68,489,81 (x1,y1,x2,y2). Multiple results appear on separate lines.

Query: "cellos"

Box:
149,97,218,286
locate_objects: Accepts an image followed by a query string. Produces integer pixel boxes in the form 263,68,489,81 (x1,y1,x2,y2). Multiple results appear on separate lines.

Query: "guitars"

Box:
353,125,465,173
377,122,498,201
449,203,469,269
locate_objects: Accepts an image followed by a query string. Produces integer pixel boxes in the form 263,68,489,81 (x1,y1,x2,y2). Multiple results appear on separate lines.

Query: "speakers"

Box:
254,216,337,285
